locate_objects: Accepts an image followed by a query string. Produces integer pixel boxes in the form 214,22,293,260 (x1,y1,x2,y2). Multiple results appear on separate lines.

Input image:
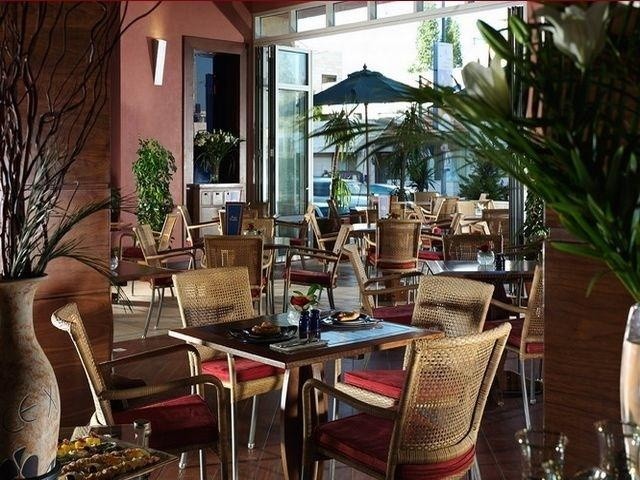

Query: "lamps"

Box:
152,38,167,86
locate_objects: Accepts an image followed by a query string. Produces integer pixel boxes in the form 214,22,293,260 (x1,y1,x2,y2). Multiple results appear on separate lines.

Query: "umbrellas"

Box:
277,64,456,213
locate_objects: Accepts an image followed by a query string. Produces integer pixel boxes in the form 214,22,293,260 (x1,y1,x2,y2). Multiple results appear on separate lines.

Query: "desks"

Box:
167,307,426,476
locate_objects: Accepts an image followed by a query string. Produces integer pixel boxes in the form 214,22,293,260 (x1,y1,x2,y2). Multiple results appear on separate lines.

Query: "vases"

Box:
210,158,221,181
0,273,62,480
619,304,640,480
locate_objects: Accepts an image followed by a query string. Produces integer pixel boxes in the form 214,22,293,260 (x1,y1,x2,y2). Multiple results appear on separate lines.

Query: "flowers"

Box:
194,129,248,172
294,0,640,304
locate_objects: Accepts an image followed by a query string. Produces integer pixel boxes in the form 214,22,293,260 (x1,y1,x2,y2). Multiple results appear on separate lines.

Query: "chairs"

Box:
342,275,496,410
301,322,513,478
480,265,544,432
169,265,287,469
111,194,504,318
50,302,229,478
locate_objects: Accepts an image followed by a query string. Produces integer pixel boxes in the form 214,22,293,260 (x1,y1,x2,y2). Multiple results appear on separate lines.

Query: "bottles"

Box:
494,252,505,269
299,311,311,339
308,309,321,340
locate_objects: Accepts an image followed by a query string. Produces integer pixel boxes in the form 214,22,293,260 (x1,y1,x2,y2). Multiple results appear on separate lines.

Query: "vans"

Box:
313,176,376,207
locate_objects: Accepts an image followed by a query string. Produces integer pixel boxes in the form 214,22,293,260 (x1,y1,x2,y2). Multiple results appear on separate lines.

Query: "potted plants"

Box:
313,110,364,261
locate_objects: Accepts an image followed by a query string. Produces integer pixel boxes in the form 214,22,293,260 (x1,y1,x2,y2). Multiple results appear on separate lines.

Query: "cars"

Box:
365,183,440,197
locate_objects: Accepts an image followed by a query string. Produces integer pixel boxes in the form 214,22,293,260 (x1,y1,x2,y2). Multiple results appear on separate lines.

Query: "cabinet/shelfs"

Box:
186,184,245,238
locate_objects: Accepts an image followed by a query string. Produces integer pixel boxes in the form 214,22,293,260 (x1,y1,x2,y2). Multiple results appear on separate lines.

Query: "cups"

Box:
512,427,570,480
592,419,640,480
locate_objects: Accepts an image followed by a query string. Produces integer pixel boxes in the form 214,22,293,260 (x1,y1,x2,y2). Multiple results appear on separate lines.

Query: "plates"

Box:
226,325,298,345
269,337,329,352
319,313,382,330
57,433,180,480
